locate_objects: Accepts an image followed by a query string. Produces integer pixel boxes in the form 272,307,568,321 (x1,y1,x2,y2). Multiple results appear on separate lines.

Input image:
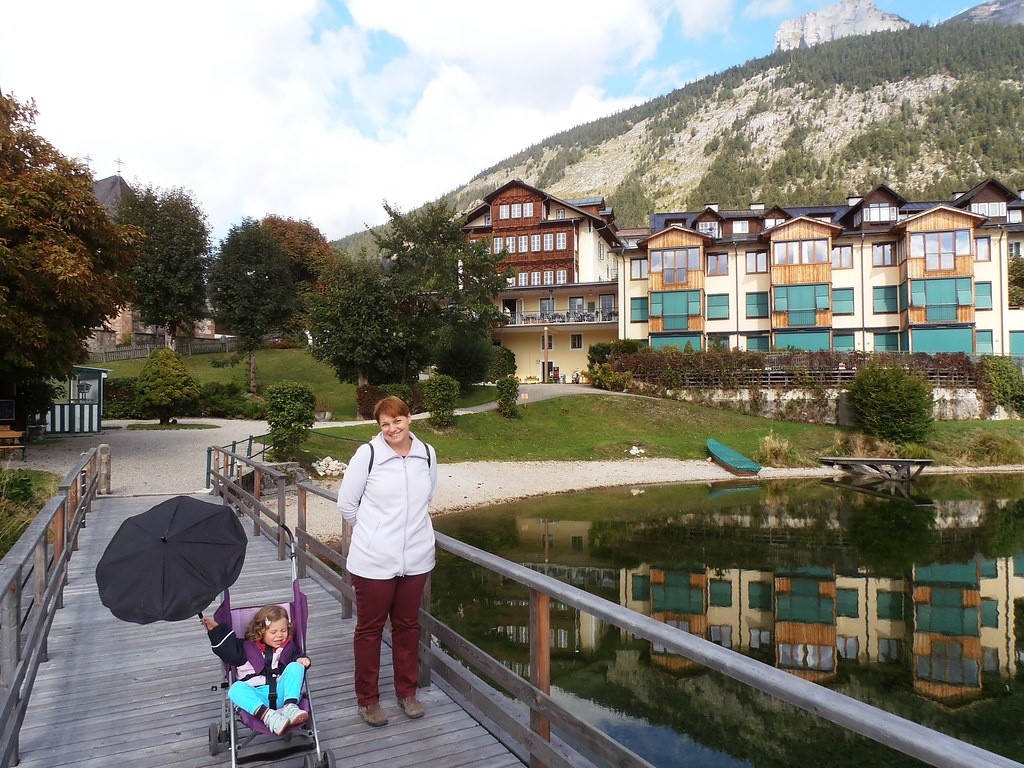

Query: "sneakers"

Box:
356,702,389,726
261,708,288,736
280,703,308,726
397,694,425,718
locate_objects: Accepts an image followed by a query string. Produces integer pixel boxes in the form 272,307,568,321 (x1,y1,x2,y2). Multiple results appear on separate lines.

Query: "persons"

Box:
504,305,511,318
336,395,438,728
199,604,312,735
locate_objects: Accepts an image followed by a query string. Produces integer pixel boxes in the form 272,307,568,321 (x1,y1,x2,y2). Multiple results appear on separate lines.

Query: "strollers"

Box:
205,522,336,768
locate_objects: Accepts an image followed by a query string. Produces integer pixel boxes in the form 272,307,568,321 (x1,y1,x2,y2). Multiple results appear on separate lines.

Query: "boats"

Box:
706,438,762,477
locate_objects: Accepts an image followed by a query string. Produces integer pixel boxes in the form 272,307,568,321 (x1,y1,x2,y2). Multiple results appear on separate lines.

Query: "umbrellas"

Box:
95,496,249,633
